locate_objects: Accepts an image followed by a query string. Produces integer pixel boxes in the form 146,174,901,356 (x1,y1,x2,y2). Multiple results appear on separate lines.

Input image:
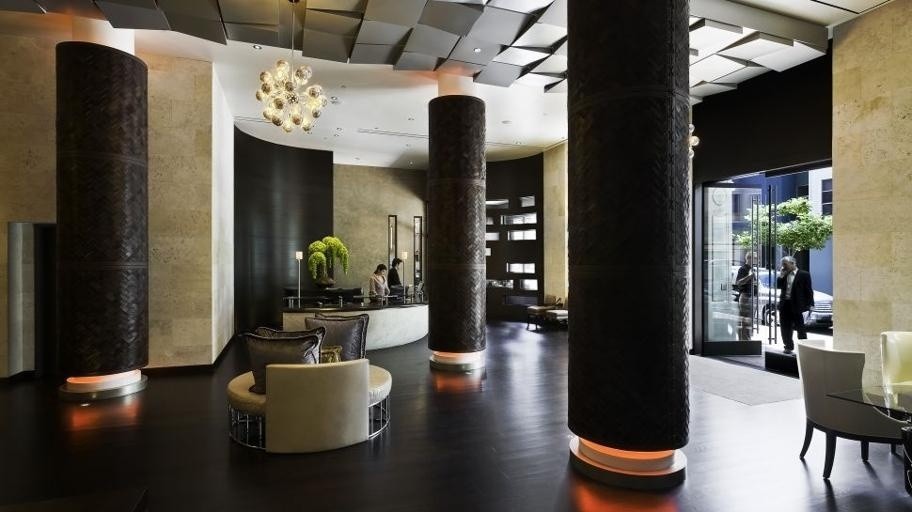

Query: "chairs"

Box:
226,356,392,453
526,295,557,331
879,330,912,454
545,297,569,331
792,337,908,482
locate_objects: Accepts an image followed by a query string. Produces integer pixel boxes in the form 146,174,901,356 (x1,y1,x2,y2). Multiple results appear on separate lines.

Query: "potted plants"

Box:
307,236,350,289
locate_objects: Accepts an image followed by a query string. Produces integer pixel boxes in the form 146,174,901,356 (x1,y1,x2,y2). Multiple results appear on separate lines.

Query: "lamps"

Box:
295,251,303,309
255,0,328,133
689,124,701,159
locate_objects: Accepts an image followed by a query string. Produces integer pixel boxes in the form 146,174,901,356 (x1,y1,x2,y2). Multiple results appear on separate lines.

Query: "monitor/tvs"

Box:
416,281,424,291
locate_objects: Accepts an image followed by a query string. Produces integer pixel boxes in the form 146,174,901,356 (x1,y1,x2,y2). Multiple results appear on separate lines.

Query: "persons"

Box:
735,251,760,342
387,257,403,288
772,255,814,354
369,264,391,306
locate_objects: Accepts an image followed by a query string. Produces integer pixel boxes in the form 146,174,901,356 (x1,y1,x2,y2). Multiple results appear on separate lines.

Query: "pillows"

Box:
239,313,370,394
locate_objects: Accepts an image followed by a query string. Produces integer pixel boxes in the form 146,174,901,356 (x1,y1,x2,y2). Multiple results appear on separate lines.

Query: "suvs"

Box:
731,264,834,329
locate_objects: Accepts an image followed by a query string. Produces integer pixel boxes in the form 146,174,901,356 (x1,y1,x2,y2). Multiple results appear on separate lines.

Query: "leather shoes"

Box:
783,348,791,354
